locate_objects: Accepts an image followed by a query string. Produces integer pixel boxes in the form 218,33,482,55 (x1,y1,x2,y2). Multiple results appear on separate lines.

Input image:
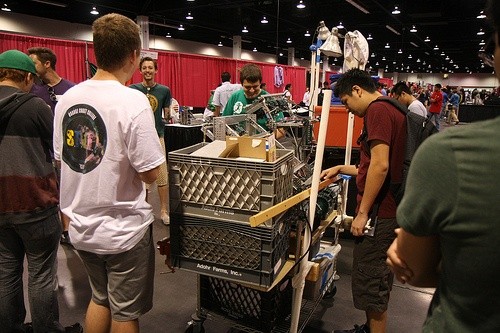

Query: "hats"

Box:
0,49,43,84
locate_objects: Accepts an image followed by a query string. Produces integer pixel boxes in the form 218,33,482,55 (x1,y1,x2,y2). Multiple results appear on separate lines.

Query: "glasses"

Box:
47,86,56,103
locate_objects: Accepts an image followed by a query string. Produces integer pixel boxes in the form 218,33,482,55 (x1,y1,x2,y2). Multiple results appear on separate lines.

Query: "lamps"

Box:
1,0,488,75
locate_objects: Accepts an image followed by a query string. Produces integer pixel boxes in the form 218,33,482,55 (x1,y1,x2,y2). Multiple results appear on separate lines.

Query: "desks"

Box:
165,123,204,146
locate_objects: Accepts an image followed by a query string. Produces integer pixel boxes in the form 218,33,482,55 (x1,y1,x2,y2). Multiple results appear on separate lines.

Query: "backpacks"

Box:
357,96,440,207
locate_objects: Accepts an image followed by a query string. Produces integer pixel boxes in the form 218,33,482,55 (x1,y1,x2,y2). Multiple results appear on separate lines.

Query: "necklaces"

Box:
142,82,156,91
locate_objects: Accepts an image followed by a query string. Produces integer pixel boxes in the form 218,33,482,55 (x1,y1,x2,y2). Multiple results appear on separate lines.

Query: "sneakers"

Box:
65,322,83,333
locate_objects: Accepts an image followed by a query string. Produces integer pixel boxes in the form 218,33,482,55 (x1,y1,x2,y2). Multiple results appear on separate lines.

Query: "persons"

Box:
203,83,222,120
52,12,166,333
222,63,286,141
319,67,415,333
26,47,78,113
298,77,500,131
211,71,244,117
386,116,499,333
260,83,267,91
282,83,295,108
1,49,84,333
126,56,172,225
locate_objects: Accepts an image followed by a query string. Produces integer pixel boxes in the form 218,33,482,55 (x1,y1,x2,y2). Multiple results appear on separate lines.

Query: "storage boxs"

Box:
302,240,342,300
313,105,365,148
167,213,291,286
200,274,292,332
167,141,295,228
